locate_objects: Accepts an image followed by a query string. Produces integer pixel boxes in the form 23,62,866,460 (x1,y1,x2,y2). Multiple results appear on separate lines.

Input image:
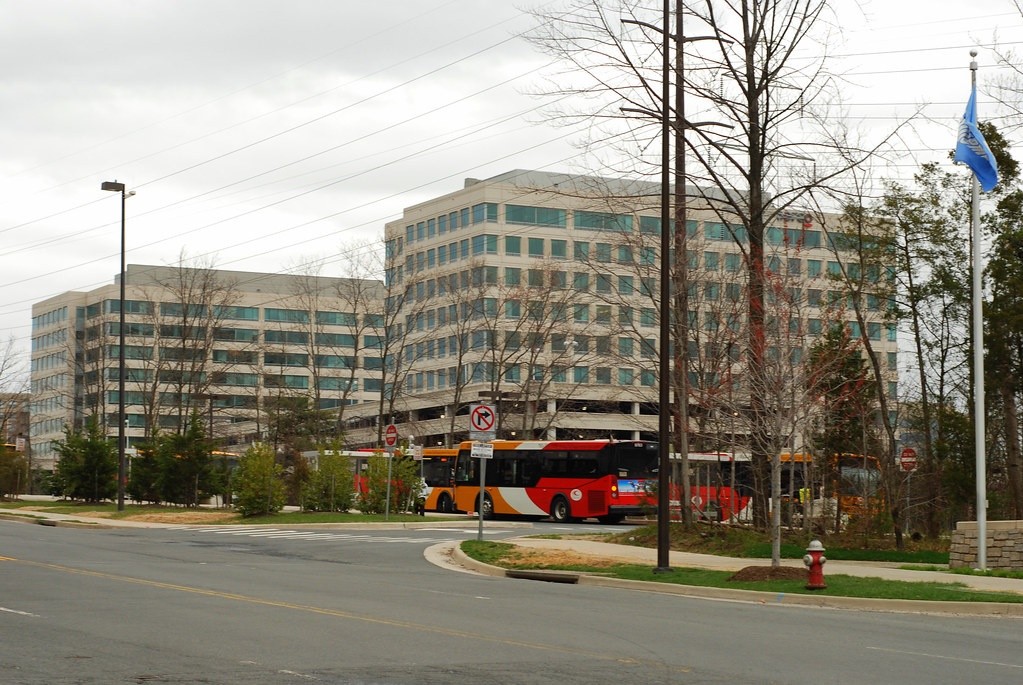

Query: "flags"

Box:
955,91,997,193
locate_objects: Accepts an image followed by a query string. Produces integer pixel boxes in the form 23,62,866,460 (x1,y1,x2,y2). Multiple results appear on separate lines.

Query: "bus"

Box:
393,447,474,514
650,450,760,527
452,438,662,525
767,453,889,537
354,447,410,514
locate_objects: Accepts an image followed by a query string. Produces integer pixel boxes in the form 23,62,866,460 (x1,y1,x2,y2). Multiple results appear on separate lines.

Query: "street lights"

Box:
99,180,130,512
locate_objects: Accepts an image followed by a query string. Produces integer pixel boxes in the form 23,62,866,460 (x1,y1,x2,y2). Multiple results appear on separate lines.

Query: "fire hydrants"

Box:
802,539,830,589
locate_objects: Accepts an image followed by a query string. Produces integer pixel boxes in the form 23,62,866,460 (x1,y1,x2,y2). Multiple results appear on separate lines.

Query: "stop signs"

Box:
385,425,397,446
899,446,919,471
